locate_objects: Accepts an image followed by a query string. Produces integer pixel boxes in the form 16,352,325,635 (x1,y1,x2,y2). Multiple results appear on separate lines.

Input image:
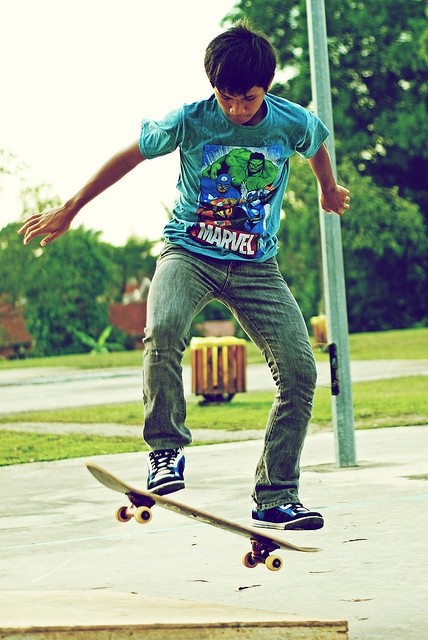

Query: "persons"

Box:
18,25,352,533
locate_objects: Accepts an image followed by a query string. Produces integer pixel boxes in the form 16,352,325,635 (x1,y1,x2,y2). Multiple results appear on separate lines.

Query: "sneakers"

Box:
147,446,185,496
253,503,324,530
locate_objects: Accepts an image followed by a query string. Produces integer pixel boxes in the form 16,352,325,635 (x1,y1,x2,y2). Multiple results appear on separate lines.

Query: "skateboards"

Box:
86,464,320,572
